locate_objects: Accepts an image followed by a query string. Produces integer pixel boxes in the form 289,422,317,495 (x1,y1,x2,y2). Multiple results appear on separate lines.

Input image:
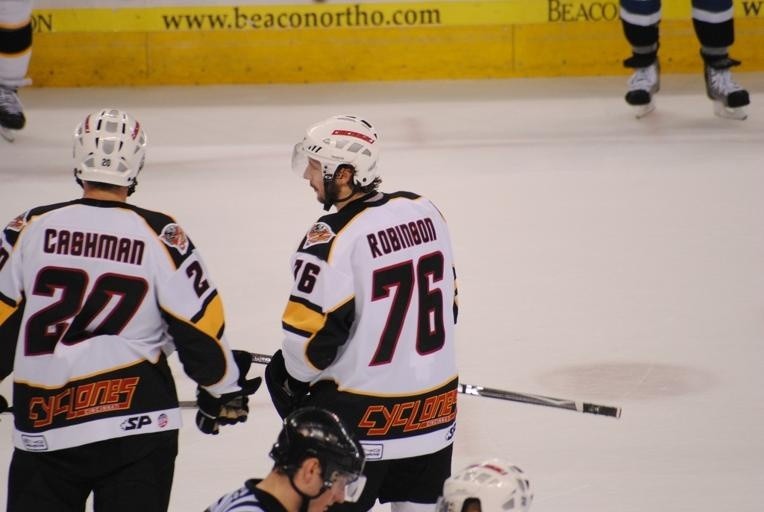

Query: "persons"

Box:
0,1,35,143
202,407,365,511
0,107,249,510
434,457,533,510
619,1,751,122
265,115,458,510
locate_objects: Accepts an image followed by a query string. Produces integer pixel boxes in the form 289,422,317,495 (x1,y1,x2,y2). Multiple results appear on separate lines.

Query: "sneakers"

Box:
704,65,750,108
1,85,28,131
624,62,659,106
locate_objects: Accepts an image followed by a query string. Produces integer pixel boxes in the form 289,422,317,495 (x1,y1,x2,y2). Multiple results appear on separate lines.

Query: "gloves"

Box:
195,387,248,436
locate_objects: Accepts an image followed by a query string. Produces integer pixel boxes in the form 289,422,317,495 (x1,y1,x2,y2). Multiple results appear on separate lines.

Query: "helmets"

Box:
71,108,146,195
268,407,365,485
443,462,530,512
292,113,381,188
